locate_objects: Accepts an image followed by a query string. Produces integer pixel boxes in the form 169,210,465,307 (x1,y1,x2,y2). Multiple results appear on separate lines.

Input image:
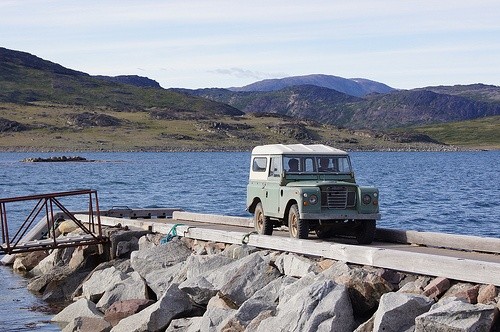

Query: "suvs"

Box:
246,144,381,246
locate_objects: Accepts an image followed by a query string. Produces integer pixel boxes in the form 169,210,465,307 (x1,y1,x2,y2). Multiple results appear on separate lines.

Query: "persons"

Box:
287,159,301,172
315,159,340,172
